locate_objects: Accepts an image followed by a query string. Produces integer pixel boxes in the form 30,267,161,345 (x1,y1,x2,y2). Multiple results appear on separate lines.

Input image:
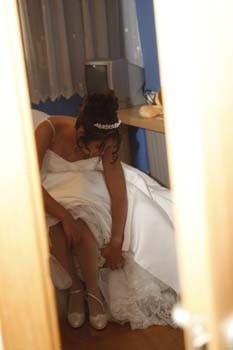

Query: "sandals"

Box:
67,287,85,328
85,289,109,330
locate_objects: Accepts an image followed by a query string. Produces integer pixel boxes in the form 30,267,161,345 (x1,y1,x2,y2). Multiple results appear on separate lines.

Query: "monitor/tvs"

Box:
82,58,144,109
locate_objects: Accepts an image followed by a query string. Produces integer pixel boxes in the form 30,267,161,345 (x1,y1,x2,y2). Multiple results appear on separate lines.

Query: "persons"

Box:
35,89,182,330
